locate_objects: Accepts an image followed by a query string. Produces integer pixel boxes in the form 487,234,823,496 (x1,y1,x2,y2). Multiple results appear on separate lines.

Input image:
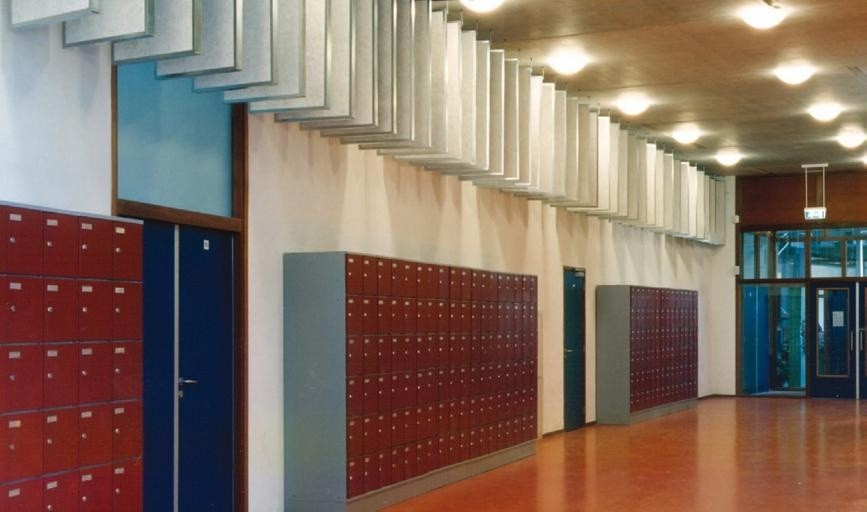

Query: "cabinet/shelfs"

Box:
283,252,537,512
0,202,143,512
596,285,698,423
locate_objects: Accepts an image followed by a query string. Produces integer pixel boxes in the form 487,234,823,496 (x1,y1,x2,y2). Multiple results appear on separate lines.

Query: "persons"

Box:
781,350,789,388
